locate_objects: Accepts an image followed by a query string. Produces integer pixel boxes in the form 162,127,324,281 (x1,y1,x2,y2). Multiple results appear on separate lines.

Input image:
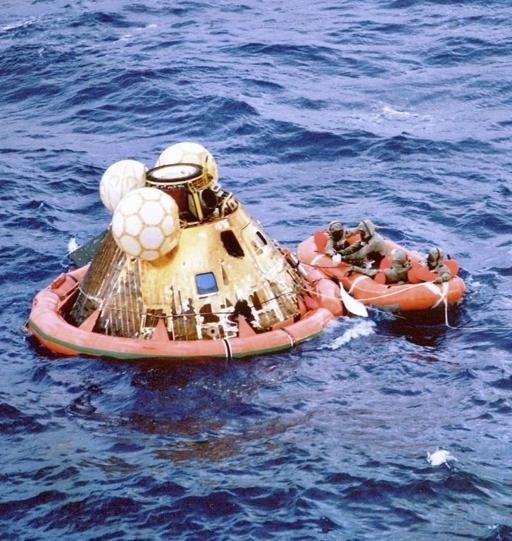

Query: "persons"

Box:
323,218,412,286
426,248,452,284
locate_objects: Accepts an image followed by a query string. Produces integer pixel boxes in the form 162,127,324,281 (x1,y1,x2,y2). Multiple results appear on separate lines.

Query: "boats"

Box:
295,226,468,312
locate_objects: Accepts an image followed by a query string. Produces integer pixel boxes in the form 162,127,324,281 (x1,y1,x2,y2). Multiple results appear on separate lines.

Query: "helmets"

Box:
357,220,376,239
428,247,443,267
328,221,345,238
391,249,408,265
